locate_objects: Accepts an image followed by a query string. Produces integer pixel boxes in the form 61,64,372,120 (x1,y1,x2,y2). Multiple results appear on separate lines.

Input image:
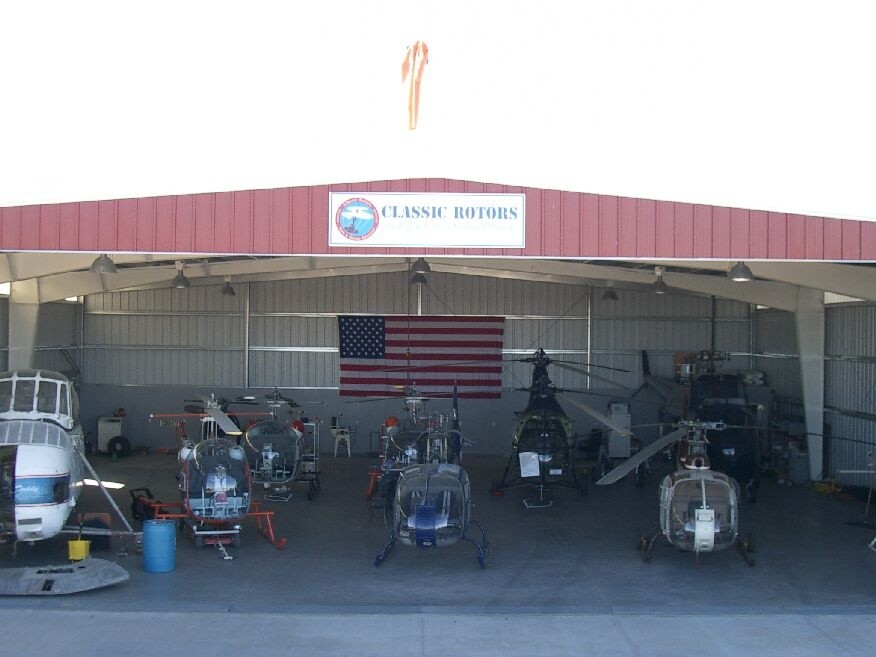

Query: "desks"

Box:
747,383,776,434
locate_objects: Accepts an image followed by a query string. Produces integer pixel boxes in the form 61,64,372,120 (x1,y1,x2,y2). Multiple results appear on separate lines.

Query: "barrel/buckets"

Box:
143,519,177,573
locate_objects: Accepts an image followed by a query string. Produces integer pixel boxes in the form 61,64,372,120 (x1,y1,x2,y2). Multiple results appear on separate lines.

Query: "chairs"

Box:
710,497,729,532
433,488,457,519
670,501,689,529
405,489,422,516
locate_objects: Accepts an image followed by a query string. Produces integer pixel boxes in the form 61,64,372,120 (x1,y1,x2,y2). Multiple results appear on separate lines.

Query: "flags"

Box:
336,314,506,400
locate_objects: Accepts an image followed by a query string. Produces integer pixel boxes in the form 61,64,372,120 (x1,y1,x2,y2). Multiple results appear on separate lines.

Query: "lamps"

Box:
409,257,431,273
650,266,669,294
407,273,428,283
169,262,191,289
222,276,236,296
89,254,118,274
602,280,619,300
725,260,755,282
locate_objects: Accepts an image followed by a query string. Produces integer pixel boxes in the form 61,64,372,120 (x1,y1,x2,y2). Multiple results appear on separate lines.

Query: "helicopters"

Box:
377,348,638,510
0,368,143,553
150,391,323,550
341,389,490,568
595,344,875,570
